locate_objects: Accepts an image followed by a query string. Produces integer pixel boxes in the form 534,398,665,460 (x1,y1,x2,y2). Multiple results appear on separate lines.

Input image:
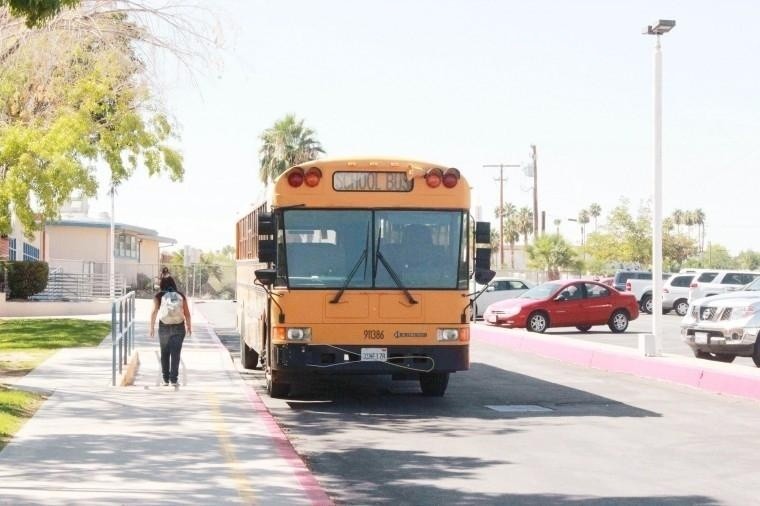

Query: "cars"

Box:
469,270,760,332
679,275,760,368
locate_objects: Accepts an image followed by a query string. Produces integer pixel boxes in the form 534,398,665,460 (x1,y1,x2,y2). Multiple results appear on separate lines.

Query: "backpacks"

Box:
158,292,184,326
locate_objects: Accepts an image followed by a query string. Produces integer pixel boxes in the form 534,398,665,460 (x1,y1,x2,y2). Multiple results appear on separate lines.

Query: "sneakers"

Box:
163,381,177,386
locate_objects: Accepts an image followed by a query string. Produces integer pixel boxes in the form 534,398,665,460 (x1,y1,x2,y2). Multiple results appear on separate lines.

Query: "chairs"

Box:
558,288,608,300
280,222,446,286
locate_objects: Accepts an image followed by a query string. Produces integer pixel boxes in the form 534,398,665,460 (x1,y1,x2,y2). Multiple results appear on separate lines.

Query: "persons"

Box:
151,274,194,389
155,267,172,289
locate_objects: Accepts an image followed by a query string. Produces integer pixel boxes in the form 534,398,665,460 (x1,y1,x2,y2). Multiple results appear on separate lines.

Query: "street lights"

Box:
642,20,677,356
483,163,520,270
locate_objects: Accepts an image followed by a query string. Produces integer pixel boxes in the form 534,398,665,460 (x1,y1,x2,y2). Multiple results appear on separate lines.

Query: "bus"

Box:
238,156,496,397
